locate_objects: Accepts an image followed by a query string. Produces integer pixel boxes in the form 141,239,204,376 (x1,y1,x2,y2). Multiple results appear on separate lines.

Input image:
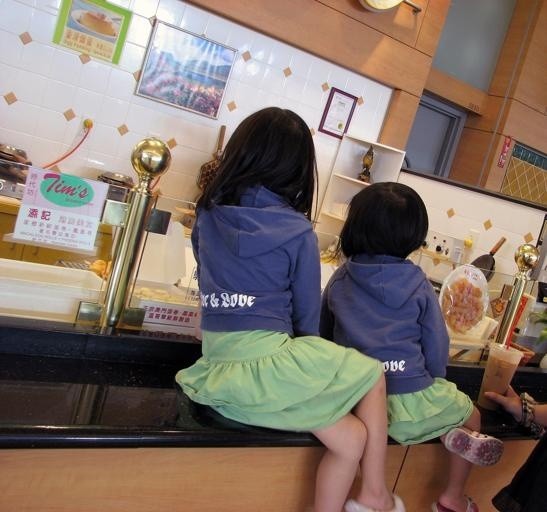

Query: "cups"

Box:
477,342,525,411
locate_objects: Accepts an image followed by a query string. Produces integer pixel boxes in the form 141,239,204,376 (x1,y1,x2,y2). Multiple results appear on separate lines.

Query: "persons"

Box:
319,181,504,512
485,385,547,512
173,106,407,512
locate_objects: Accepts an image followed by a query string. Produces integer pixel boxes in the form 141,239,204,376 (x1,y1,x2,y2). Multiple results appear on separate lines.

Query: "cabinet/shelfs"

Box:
314,135,407,233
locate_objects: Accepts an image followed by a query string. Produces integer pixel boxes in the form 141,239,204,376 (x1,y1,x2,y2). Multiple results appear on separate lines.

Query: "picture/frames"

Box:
132,17,238,120
318,86,359,138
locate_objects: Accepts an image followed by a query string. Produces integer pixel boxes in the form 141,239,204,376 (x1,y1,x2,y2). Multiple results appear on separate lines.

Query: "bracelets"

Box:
515,392,536,429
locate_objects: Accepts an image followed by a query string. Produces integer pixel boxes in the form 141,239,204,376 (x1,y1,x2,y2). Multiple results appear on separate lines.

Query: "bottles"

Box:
358,146,373,182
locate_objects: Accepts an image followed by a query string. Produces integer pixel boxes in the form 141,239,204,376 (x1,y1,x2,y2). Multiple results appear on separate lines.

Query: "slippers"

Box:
432,495,479,512
443,427,504,466
345,494,406,512
